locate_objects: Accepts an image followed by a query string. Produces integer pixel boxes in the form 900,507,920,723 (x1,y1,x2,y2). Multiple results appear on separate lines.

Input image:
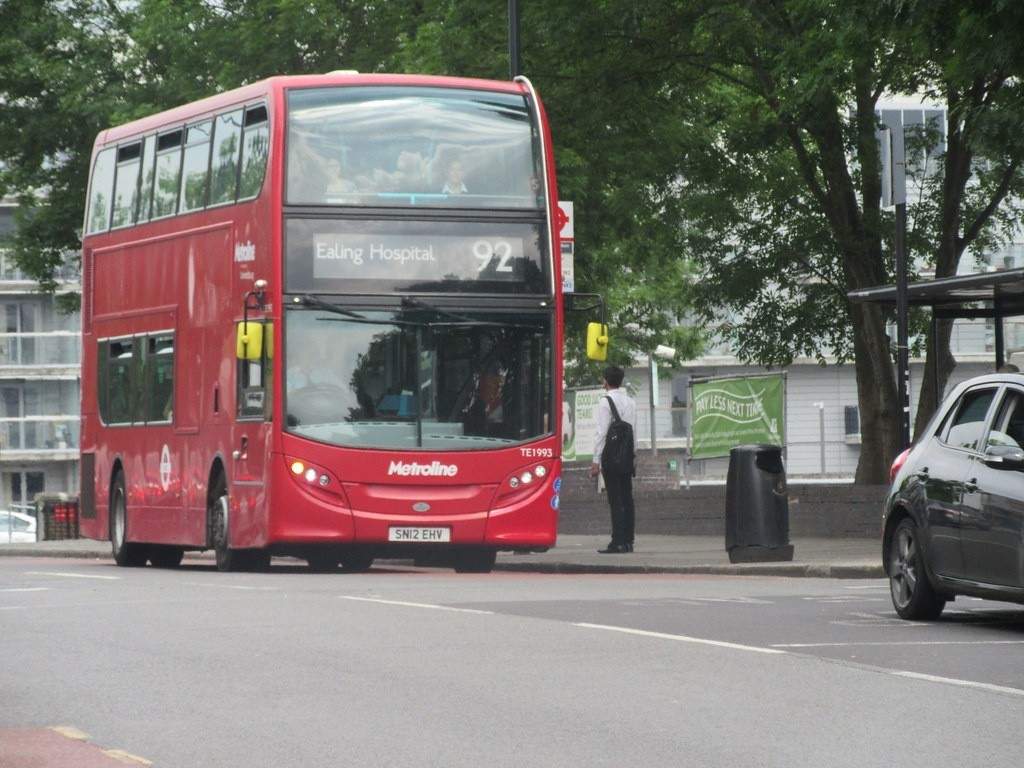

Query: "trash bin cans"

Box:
725,445,794,564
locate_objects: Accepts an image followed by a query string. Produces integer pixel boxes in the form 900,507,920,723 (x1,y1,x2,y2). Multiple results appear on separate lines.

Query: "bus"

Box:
73,70,611,580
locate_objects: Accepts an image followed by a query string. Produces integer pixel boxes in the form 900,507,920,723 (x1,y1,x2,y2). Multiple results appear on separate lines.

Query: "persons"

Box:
463,368,535,443
288,133,486,209
286,336,359,425
589,363,637,556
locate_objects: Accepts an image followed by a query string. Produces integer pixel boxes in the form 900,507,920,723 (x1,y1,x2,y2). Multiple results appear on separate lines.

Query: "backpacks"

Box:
600,396,635,476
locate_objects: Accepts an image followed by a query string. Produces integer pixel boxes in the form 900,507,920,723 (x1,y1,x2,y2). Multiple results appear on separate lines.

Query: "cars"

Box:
875,373,1024,620
